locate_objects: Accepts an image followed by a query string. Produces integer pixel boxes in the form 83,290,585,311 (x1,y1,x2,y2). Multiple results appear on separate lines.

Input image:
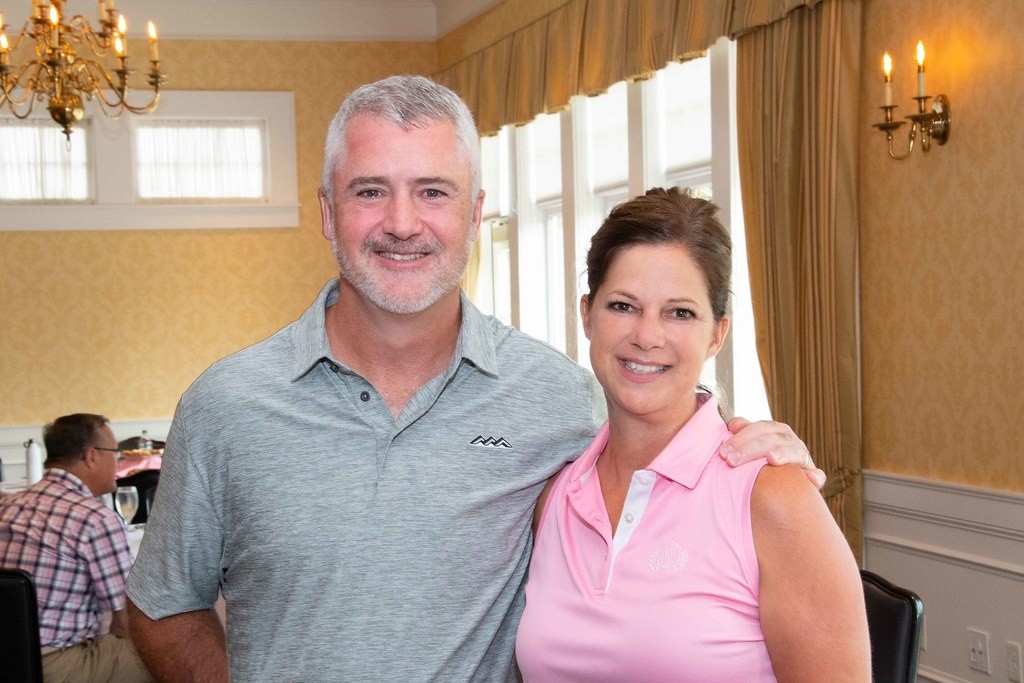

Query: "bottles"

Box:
138,430,152,451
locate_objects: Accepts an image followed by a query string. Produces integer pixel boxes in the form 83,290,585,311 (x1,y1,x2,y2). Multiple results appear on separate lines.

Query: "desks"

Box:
0,477,27,495
124,522,227,636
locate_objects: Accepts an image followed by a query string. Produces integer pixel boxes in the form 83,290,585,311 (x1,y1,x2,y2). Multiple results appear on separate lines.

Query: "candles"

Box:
882,53,893,106
915,41,926,96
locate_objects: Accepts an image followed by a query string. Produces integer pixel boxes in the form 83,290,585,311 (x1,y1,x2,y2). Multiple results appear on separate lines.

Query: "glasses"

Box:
93,447,121,459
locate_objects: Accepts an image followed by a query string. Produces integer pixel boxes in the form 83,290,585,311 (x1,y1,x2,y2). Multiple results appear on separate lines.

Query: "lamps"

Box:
0,0,166,142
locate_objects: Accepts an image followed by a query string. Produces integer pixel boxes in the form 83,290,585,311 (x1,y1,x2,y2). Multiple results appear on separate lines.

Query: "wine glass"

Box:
115,486,139,531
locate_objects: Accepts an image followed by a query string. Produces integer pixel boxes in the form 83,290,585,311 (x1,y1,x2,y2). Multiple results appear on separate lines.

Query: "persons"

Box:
121,75,826,683
515,186,871,683
0,413,153,683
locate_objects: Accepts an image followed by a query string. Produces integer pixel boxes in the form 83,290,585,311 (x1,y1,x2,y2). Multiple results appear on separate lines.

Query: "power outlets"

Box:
1007,641,1023,683
919,615,927,651
968,628,990,674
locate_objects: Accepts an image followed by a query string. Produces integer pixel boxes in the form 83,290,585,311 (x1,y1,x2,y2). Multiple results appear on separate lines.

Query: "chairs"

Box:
0,565,44,683
112,436,166,523
857,570,923,683
